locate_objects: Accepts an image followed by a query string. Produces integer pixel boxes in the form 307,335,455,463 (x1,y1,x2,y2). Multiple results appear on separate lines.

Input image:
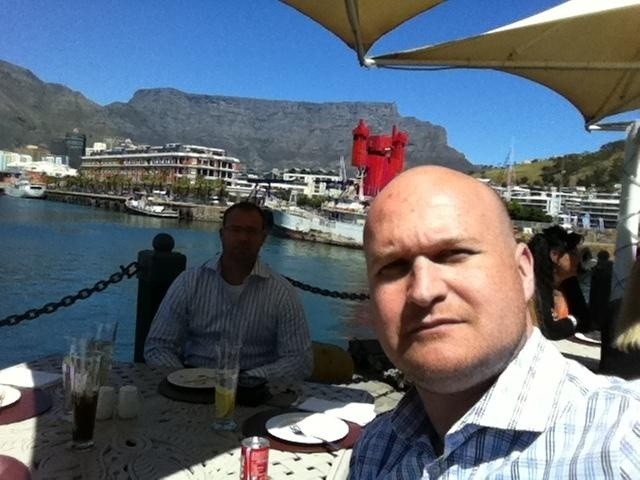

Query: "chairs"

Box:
307,341,357,385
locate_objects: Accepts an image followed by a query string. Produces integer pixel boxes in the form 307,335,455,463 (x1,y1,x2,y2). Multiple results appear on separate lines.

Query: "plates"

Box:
264,410,350,446
0,384,23,409
165,366,216,391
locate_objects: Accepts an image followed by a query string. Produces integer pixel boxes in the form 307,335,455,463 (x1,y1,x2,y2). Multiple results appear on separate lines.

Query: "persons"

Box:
348,163,640,479
143,200,315,385
522,223,584,340
577,245,594,272
589,251,613,301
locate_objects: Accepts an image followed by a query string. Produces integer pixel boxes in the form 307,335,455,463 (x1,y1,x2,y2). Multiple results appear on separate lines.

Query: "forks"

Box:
287,423,342,452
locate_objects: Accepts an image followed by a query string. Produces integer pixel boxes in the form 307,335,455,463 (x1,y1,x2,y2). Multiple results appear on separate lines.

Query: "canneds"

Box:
240,436,270,480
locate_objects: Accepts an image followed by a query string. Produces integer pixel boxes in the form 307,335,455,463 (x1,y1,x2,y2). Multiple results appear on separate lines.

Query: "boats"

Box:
274,198,364,246
124,197,179,218
5,179,45,198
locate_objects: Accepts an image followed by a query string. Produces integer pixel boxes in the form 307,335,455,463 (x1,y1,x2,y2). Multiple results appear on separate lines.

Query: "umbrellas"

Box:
282,0,446,64
360,1,640,126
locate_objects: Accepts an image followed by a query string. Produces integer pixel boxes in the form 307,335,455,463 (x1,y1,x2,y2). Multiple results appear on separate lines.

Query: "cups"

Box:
210,338,243,432
117,385,140,419
55,315,119,451
